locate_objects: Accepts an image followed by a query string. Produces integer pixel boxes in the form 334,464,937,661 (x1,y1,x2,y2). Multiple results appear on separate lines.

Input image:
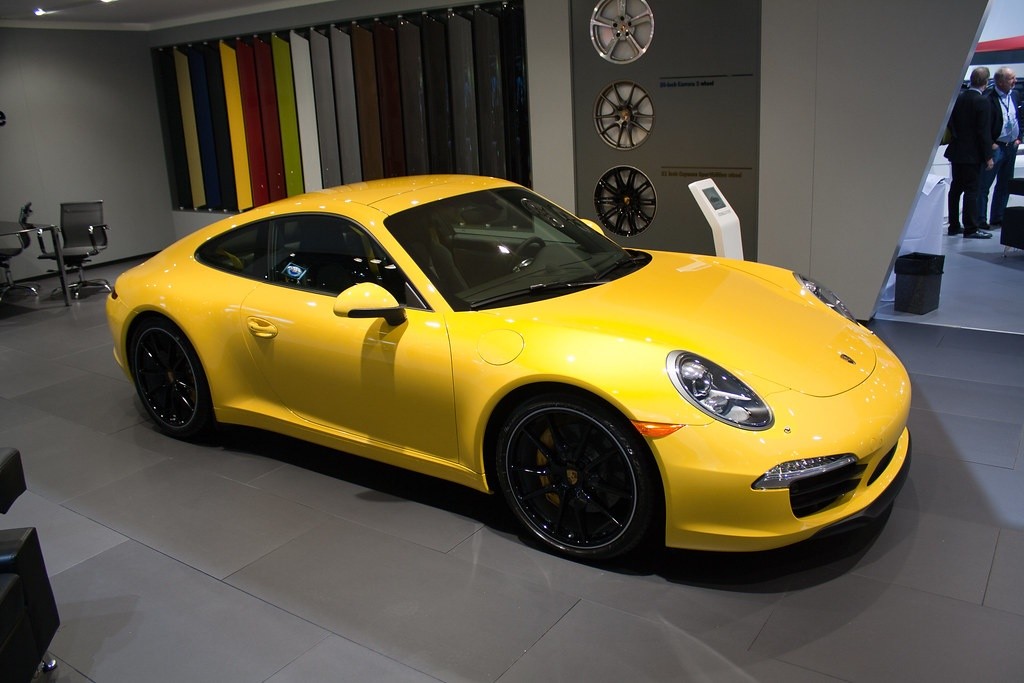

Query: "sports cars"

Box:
105,169,912,562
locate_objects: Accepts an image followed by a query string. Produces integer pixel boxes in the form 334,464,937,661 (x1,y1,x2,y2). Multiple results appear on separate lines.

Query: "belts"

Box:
995,140,1015,147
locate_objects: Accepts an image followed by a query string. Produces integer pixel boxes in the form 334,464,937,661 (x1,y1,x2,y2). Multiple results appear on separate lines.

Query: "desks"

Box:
0,222,54,320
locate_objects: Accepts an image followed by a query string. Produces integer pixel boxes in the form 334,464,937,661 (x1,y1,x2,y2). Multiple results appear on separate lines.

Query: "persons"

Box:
943,66,1024,238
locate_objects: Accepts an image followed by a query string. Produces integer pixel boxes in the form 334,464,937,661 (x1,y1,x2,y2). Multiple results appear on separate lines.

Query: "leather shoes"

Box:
963,229,992,238
948,226,965,236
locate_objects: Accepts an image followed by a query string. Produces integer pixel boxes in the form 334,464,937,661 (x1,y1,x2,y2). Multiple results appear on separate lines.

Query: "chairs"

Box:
37,199,112,299
0,202,41,301
399,219,469,308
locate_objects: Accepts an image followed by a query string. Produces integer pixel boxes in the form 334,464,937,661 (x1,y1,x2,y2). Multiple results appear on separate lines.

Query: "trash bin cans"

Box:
894,251,945,315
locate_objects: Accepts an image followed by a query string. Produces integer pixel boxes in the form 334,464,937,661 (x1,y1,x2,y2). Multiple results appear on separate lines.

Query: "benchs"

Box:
0,449,62,683
1000,177,1024,258
